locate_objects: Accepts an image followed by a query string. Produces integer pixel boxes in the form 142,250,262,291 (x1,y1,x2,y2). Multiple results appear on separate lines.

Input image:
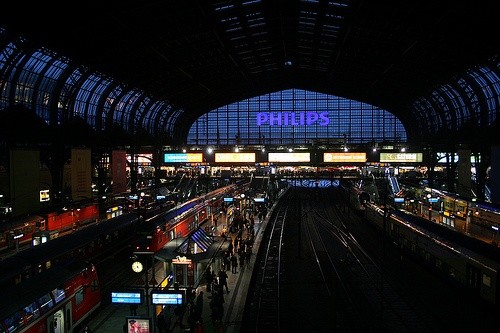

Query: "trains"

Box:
349,184,500,312
1,183,237,333
0,179,201,256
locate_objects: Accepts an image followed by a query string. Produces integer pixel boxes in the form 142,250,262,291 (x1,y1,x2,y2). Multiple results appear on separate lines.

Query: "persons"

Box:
0,163,500,333
